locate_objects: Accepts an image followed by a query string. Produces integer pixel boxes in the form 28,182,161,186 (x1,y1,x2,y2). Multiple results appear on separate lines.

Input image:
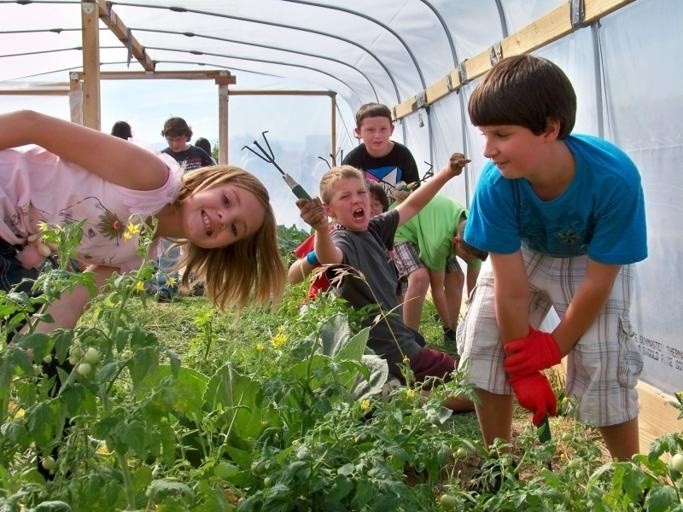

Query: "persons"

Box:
449,52,652,493
1,109,290,485
151,113,216,300
390,192,487,342
194,134,219,166
110,120,134,141
294,150,475,417
288,179,391,300
338,101,421,307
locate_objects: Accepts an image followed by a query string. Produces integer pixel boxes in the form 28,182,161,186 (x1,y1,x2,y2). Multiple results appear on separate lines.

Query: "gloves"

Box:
502,323,562,379
509,371,559,426
